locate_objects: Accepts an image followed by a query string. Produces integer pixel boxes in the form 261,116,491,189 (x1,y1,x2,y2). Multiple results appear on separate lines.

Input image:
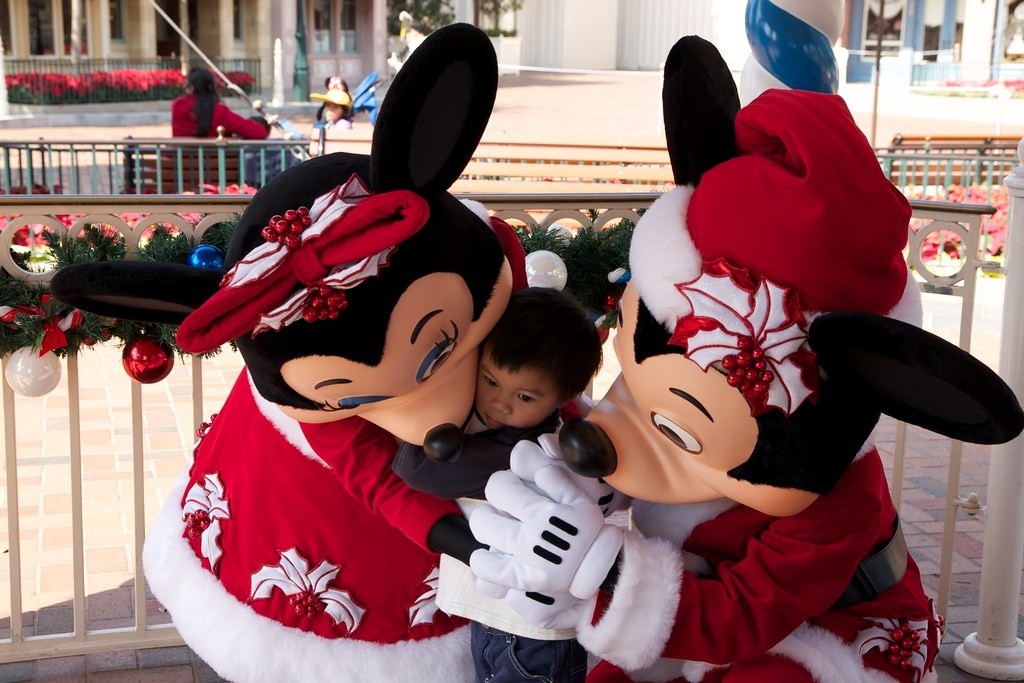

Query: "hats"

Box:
309,89,352,110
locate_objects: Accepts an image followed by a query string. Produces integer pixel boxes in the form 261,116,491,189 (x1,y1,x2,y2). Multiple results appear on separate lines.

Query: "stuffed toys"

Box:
469,33,1024,682
49,23,631,683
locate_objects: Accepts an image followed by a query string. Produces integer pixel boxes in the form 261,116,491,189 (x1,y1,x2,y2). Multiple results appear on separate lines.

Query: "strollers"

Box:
245,70,386,187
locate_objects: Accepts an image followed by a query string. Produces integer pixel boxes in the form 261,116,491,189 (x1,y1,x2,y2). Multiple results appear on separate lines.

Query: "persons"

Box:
389,285,601,683
170,66,272,139
308,88,354,131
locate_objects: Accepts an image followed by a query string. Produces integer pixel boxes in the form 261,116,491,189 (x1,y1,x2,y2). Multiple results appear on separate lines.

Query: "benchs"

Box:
123,136,310,195
874,134,1023,188
